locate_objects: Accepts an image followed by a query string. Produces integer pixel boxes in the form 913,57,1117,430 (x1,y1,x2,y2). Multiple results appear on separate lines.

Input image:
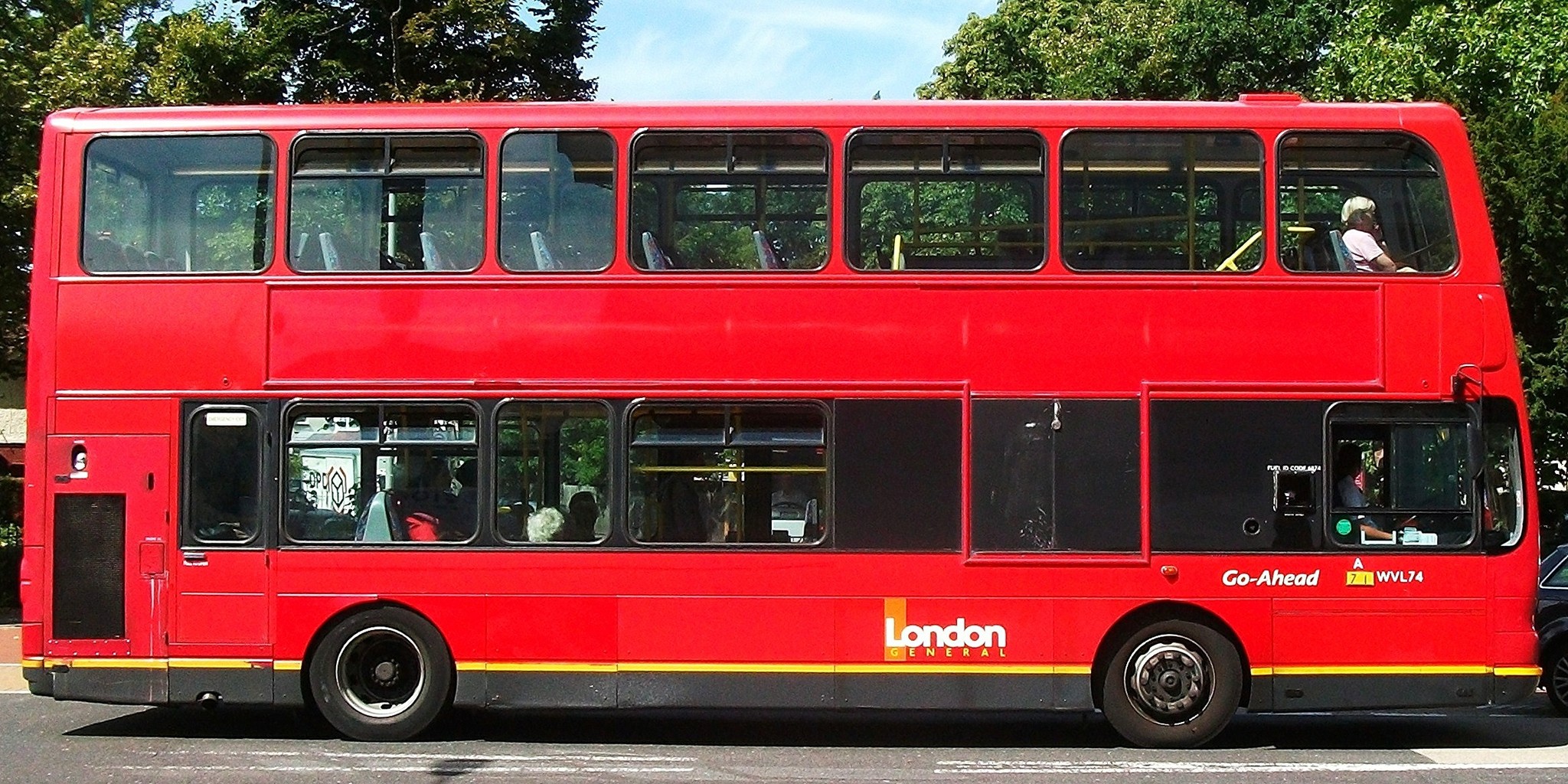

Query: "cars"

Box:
1533,544,1568,705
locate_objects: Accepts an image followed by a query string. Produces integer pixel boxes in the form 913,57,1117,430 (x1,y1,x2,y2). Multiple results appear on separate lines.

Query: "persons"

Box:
0,507,26,613
192,437,817,540
1333,443,1398,539
1339,197,1424,274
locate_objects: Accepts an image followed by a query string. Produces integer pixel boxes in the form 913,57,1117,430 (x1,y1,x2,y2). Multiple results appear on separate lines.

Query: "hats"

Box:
1341,196,1376,223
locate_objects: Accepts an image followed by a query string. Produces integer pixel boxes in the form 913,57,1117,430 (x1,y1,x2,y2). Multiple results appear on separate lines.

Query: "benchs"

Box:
354,488,411,545
1298,231,1357,271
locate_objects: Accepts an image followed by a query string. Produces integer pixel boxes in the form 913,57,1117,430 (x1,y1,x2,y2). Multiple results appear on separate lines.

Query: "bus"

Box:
16,95,1546,750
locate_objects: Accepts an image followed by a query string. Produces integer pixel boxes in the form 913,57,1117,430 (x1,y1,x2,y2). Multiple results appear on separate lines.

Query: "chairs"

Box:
367,244,402,269
319,231,351,271
802,498,822,542
529,230,560,269
641,231,667,269
92,233,184,271
752,229,783,268
892,233,906,270
419,231,457,270
